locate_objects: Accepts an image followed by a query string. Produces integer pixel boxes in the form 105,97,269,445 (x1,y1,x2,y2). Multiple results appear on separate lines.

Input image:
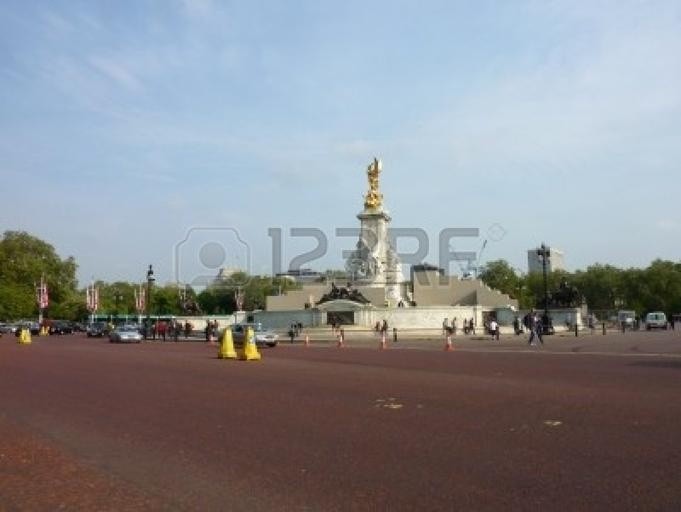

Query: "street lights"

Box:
537,241,556,337
144,263,155,341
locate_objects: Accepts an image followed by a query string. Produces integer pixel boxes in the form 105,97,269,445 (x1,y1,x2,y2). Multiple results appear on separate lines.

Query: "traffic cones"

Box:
216,327,238,359
338,335,344,349
443,331,456,350
240,327,263,362
304,335,309,346
378,329,388,351
18,328,33,343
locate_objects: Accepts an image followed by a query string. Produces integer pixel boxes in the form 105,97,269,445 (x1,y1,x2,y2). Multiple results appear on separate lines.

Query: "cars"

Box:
218,322,280,347
1,317,108,337
109,324,143,344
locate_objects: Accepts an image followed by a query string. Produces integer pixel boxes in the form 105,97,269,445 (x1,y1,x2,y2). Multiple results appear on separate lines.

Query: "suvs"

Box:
617,310,637,327
645,312,667,330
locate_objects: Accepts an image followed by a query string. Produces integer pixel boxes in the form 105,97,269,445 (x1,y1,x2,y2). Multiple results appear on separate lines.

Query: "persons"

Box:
141,314,479,343
526,311,544,345
489,318,499,340
